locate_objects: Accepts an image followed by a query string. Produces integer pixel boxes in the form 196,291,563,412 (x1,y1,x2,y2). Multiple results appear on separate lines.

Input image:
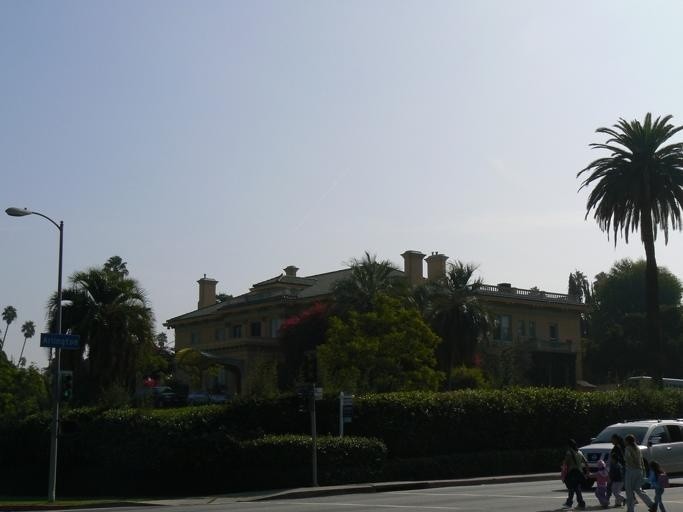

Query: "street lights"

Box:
6,208,63,504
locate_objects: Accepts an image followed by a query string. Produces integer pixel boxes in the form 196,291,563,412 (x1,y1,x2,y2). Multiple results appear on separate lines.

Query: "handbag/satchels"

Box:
568,467,587,487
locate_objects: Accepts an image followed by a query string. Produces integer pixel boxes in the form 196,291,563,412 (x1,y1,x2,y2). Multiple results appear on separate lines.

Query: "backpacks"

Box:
658,471,670,488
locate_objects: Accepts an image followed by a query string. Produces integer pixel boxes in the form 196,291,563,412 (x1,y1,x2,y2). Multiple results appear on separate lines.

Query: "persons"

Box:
642,457,668,512
559,437,590,510
585,459,609,508
622,434,654,512
607,432,626,509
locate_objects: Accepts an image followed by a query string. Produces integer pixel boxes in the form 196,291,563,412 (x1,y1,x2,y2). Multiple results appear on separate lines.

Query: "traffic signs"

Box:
40,333,82,351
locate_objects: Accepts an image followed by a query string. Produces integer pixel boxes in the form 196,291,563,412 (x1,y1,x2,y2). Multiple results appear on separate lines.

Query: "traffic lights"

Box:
59,371,73,402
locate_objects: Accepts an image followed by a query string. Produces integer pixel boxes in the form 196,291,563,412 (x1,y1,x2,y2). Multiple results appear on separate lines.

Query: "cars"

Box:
151,386,185,408
576,417,683,489
